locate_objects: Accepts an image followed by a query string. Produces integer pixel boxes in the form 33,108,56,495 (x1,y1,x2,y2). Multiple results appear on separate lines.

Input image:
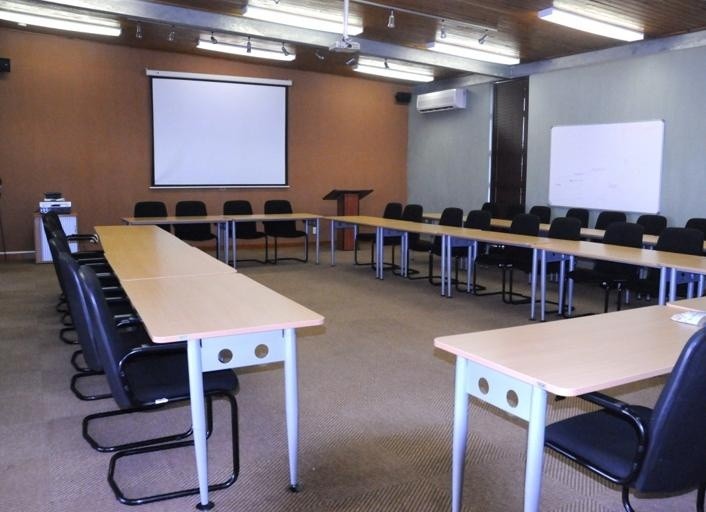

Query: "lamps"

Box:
0,0,706,79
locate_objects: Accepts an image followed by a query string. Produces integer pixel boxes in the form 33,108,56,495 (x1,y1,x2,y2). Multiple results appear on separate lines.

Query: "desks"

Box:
380,221,706,320
433,305,706,512
420,212,705,301
223,213,323,269
120,272,325,512
323,216,410,279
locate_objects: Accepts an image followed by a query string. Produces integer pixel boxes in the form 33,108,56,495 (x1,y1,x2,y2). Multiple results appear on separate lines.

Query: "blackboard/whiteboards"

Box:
547,119,666,215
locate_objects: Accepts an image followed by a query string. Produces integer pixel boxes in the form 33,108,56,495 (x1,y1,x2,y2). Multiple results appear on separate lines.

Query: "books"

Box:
670,312,705,326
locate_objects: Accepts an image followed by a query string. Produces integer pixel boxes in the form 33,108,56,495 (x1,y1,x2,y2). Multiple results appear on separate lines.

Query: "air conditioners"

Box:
416,88,467,114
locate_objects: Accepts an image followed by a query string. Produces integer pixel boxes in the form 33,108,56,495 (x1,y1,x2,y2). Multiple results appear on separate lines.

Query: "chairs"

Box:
41,211,240,505
473,213,581,313
355,203,423,275
544,327,706,512
411,208,490,293
562,222,705,319
135,200,308,265
482,204,704,241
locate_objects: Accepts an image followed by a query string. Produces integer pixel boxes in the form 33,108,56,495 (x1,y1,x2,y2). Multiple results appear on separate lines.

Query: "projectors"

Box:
329,42,360,53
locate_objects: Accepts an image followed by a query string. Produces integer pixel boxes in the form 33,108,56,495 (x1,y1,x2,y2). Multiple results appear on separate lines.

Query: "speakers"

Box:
395,92,411,103
0,57,11,73
